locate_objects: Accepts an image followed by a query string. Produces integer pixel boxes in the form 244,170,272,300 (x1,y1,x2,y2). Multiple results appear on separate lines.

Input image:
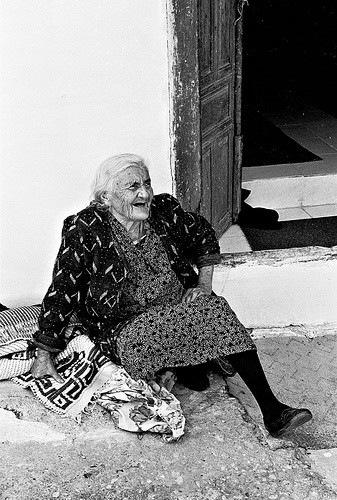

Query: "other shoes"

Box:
262,407,313,437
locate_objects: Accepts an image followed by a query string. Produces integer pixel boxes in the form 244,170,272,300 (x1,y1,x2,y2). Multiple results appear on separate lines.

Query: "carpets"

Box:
238,215,337,252
241,80,324,167
221,333,337,450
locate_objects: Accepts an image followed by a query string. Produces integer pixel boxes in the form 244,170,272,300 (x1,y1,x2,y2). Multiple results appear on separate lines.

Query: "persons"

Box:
30,154,315,438
